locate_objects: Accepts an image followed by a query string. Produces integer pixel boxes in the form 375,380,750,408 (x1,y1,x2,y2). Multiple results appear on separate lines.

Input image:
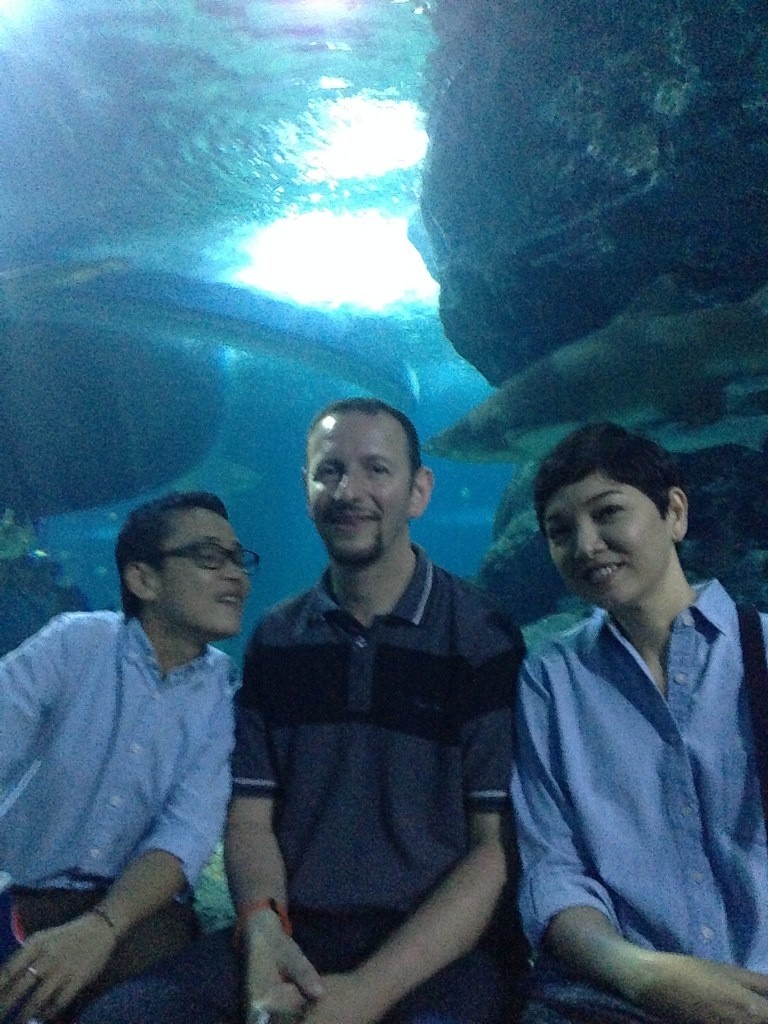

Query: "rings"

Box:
26,965,45,982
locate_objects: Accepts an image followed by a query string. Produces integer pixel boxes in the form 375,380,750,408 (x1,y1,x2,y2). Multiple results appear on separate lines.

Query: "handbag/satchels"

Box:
9,892,198,984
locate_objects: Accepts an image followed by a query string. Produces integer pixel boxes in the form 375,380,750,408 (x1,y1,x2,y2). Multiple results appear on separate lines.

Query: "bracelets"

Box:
88,907,125,947
232,896,293,961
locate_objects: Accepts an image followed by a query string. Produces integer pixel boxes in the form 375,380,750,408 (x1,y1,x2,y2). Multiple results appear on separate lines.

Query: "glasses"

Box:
160,541,260,576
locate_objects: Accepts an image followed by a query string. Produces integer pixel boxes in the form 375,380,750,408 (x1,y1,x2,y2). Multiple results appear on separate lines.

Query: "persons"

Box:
504,420,768,1024
80,400,533,1024
0,491,262,1022
